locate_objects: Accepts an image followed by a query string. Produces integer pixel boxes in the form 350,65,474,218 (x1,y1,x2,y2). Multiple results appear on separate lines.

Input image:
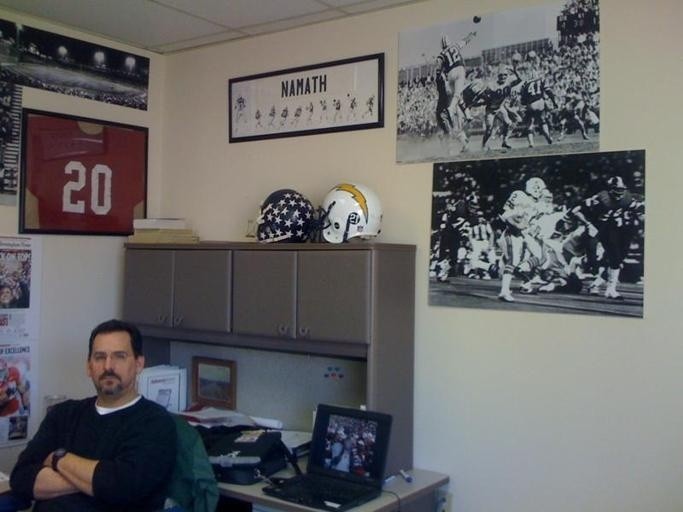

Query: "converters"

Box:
271,472,297,485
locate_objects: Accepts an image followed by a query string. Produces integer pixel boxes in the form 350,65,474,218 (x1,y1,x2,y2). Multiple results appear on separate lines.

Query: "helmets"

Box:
526,175,546,197
442,34,450,48
257,181,382,244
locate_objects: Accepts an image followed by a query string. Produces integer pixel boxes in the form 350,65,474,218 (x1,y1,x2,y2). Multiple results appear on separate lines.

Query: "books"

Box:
136,362,313,458
127,216,200,245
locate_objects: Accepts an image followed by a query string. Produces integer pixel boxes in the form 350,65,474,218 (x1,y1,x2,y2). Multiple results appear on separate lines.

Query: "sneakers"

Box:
498,291,513,302
588,283,620,299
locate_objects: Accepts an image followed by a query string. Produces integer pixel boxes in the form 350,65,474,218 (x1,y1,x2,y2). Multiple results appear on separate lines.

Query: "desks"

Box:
216,428,450,512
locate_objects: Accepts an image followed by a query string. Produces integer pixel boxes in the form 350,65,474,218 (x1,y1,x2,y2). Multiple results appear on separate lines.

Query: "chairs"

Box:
161,413,189,512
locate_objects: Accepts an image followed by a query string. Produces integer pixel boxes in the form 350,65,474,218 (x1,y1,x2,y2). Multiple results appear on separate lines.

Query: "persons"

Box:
322,417,376,477
234,93,376,131
0,54,147,195
432,153,646,302
396,0,599,153
0,251,31,309
8,318,172,512
0,359,29,417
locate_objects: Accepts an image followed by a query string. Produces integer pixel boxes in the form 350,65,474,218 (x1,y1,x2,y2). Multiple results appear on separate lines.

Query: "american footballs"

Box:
473,16,482,23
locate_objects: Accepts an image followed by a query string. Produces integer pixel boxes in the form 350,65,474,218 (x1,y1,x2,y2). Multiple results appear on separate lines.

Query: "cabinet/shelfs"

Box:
232,250,371,344
123,250,232,332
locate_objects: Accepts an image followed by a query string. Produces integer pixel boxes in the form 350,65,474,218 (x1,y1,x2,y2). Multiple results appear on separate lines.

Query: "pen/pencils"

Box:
400,468,413,482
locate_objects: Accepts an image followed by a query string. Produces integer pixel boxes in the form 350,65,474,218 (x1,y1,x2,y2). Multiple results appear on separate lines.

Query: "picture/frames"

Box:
227,52,384,143
192,356,236,407
17,106,149,236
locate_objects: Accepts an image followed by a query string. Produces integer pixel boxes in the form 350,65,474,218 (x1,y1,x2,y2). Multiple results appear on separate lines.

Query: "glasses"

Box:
91,352,135,360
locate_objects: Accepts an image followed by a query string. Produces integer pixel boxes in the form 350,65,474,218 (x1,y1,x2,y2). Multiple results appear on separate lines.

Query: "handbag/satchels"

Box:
196,425,302,484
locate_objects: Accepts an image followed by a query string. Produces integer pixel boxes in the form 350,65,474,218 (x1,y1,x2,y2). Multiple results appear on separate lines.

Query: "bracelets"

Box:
51,447,69,472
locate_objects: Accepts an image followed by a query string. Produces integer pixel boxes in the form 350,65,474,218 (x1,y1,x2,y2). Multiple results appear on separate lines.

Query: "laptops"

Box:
262,403,391,512
